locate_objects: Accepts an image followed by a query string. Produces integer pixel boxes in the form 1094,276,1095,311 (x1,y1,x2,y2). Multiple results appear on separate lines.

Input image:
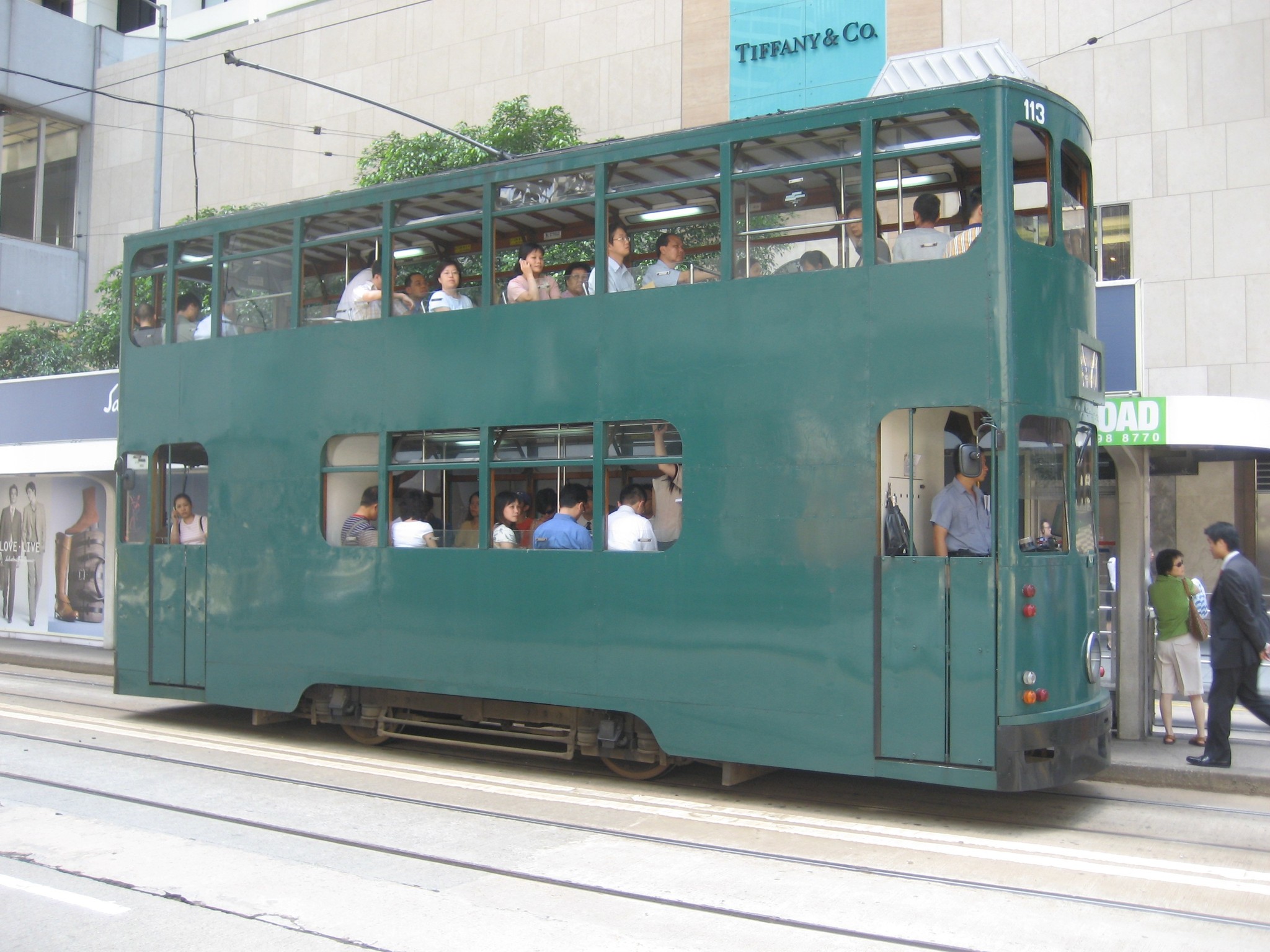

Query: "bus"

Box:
109,75,1114,798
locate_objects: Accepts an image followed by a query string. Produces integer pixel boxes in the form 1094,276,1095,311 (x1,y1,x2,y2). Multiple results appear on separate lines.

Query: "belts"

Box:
947,551,991,558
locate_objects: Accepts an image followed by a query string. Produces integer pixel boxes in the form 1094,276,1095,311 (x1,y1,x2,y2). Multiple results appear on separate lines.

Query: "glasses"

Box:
1044,526,1051,529
612,236,631,243
1172,560,1184,566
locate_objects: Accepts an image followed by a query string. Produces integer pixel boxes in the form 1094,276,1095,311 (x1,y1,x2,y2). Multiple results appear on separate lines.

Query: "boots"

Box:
54,532,79,621
65,486,99,535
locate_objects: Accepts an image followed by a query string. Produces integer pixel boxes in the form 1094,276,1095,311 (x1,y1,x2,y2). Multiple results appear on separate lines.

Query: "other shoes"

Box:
8,616,12,623
29,620,34,626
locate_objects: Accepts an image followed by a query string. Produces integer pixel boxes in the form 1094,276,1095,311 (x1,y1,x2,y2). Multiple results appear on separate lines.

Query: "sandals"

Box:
1188,735,1207,746
1164,734,1176,744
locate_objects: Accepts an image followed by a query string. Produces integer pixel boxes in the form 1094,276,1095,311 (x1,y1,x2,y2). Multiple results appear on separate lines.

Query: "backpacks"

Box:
69,529,104,621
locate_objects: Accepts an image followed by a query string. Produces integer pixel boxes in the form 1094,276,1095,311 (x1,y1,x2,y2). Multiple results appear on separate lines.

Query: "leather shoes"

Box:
1185,753,1231,767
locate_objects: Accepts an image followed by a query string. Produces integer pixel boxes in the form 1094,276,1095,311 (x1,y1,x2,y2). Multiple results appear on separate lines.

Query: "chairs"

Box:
502,288,509,306
581,279,590,296
420,289,437,313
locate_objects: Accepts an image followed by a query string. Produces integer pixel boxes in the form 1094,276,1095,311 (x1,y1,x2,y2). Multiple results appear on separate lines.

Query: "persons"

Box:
1105,558,1117,650
169,493,208,547
1184,521,1270,767
387,421,682,554
22,482,46,626
131,187,982,346
1038,519,1052,539
340,486,379,548
1147,549,1209,747
928,444,991,557
0,483,22,624
1150,546,1155,586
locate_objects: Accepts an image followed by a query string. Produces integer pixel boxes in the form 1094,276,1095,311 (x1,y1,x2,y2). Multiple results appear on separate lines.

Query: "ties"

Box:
11,508,14,521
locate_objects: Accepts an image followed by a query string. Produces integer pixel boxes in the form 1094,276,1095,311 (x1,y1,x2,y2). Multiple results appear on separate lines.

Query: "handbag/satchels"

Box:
1182,579,1208,641
